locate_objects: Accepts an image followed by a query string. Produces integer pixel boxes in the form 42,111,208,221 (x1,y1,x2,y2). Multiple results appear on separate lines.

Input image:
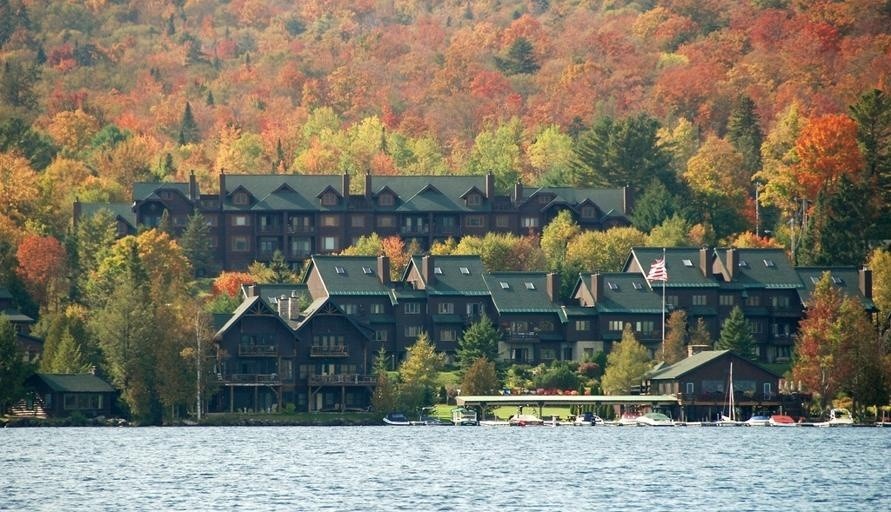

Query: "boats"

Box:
509,413,544,426
381,410,481,427
830,408,854,427
716,361,796,429
573,412,604,426
635,413,674,428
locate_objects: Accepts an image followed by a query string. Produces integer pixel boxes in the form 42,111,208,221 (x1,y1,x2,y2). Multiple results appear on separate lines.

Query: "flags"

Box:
646,256,668,282
